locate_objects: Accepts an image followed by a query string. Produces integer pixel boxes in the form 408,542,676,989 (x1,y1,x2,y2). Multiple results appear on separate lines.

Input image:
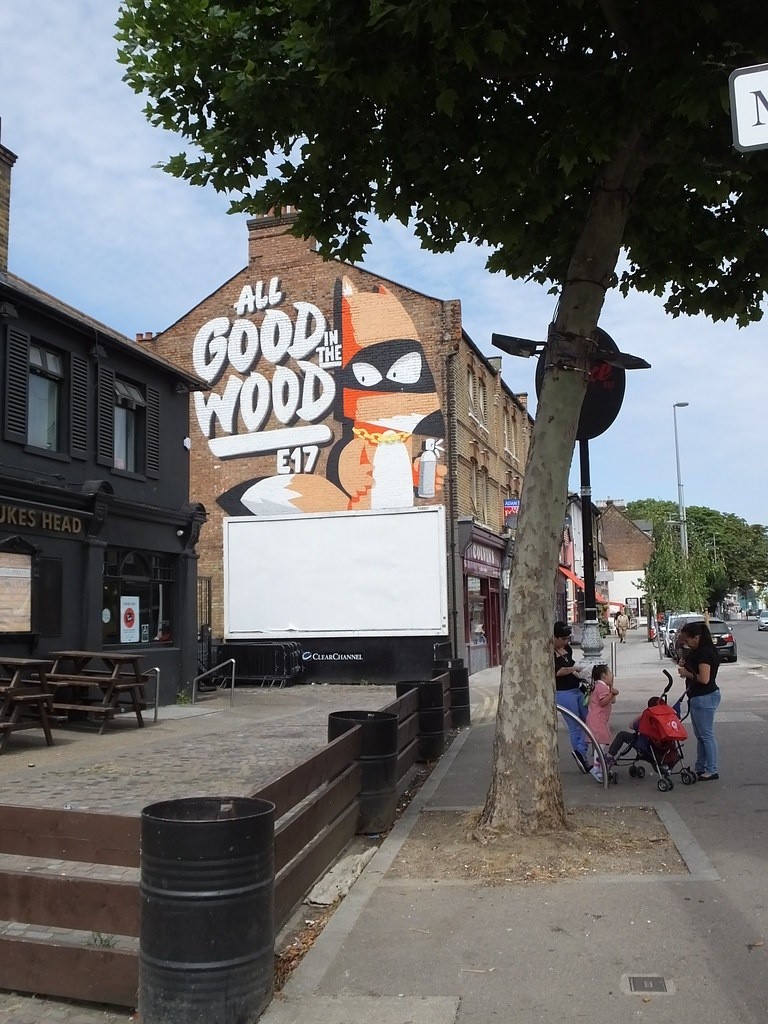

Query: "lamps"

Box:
0,301,18,319
90,343,106,359
176,382,190,393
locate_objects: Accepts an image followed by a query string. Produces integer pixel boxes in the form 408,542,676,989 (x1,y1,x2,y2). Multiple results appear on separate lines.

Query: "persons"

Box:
678,623,721,780
596,697,666,765
614,612,629,643
553,621,594,774
586,665,619,783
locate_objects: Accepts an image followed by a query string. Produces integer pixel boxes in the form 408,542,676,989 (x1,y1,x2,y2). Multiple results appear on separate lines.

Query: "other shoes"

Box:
619,639,622,642
622,641,625,643
571,749,594,774
596,753,614,765
696,770,705,776
589,767,604,784
697,771,719,781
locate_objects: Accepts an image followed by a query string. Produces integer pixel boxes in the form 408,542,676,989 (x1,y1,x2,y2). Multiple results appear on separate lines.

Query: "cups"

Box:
141,624,149,642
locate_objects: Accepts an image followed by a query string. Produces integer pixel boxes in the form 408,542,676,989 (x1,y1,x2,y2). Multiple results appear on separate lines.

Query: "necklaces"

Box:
553,647,568,662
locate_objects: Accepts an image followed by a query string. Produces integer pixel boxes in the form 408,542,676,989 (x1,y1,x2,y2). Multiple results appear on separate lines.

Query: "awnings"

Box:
559,563,609,604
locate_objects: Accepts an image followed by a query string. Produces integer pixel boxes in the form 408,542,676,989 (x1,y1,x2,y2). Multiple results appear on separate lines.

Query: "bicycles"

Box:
652,627,666,649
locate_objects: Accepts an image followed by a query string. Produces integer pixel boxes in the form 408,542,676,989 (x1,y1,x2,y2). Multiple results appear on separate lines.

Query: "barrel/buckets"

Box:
138,796,277,1024
434,658,464,668
327,711,400,834
396,681,445,762
432,668,470,726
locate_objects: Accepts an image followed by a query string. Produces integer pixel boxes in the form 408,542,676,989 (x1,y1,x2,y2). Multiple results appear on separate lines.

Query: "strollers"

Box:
606,668,698,792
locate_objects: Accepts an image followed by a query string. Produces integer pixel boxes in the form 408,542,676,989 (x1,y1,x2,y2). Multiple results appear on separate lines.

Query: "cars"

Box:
747,608,761,616
756,610,768,631
658,610,738,665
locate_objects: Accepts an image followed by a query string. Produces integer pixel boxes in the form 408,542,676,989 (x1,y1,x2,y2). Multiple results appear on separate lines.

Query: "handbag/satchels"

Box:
638,698,688,741
579,679,588,694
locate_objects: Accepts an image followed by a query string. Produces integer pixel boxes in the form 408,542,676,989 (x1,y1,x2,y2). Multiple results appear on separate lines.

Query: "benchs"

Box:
0,669,157,755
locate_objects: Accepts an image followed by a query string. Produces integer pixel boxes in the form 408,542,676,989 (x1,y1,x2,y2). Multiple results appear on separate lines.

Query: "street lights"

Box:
713,531,720,565
671,401,689,614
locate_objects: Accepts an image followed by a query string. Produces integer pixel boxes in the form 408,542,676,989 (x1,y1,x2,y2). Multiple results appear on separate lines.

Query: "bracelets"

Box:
611,692,615,696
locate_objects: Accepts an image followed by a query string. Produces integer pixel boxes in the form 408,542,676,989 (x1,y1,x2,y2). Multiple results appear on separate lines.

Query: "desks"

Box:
0,651,147,753
